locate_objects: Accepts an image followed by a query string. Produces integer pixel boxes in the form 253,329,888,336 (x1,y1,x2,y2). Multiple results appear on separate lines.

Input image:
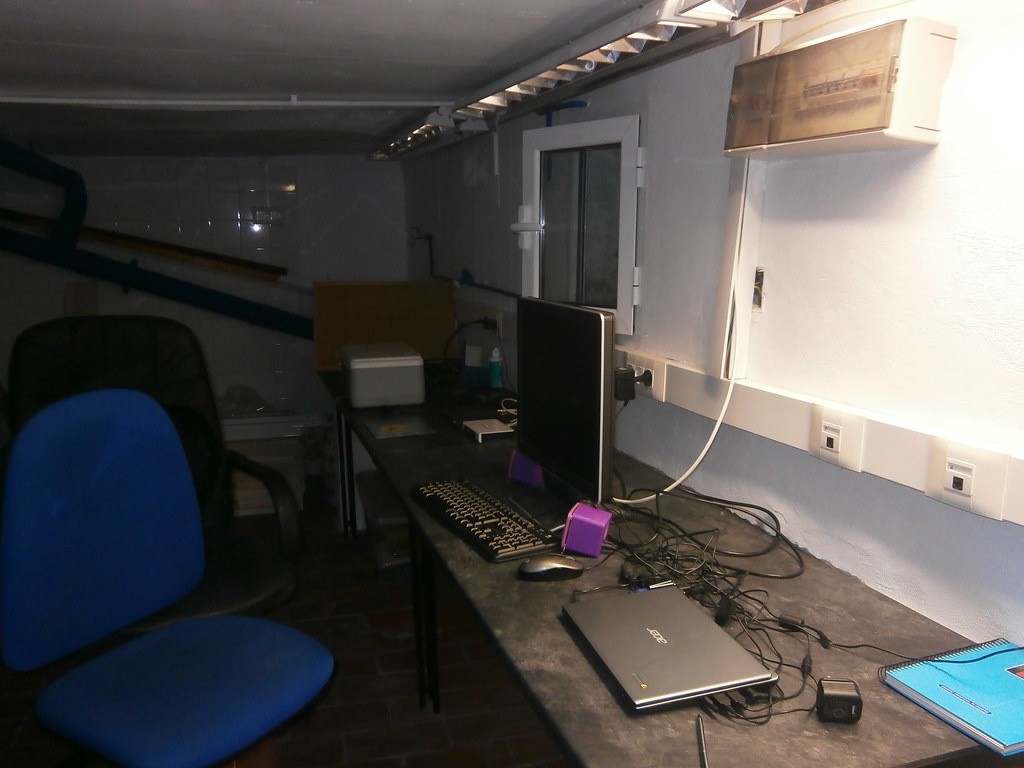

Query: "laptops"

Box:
562,586,780,715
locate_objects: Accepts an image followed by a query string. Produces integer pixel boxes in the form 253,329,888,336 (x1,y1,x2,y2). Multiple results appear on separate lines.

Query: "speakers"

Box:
560,501,612,559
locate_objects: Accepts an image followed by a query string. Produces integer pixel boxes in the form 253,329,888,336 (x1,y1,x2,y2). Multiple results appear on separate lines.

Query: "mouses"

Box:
517,553,584,581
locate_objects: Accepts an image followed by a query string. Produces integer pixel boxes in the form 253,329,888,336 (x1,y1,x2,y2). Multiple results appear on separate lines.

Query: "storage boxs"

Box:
341,340,428,409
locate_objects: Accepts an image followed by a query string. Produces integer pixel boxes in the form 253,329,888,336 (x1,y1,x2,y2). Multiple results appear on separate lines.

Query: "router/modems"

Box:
462,418,514,445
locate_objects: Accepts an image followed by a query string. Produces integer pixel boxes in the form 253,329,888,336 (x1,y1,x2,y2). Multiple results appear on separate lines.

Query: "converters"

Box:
817,677,863,725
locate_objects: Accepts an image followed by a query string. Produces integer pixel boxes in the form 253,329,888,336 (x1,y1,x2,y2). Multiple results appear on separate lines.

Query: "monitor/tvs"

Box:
508,294,616,533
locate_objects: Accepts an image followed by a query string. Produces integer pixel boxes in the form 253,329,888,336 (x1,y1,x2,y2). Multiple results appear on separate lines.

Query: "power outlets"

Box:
622,362,657,395
943,456,975,498
818,420,842,453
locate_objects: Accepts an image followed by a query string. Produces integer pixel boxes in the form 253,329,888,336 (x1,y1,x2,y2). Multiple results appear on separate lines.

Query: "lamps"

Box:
385,0,810,159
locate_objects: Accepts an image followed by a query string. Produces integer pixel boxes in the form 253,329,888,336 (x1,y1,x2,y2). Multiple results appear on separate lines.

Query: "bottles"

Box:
489,348,505,388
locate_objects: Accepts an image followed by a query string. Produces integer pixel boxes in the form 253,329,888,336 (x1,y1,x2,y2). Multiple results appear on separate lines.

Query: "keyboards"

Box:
410,475,566,564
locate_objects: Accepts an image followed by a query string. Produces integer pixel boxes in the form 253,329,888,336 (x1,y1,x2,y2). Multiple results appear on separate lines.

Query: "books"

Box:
876,636,1024,757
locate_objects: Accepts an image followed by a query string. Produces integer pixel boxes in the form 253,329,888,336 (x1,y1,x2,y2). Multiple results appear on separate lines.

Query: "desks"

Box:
318,361,1024,768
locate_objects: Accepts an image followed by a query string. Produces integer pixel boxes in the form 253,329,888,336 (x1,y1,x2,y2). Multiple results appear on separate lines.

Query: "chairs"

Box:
11,312,295,639
1,387,337,768
